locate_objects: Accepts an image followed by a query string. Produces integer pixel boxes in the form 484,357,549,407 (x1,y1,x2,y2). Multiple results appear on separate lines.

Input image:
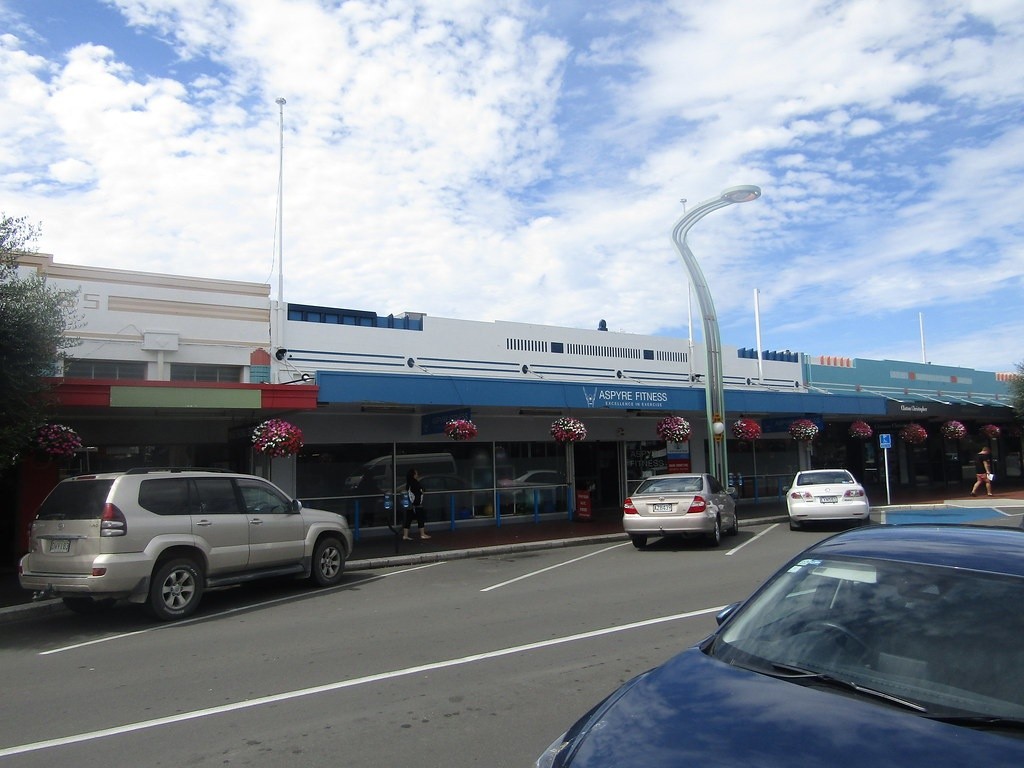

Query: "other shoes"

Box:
970,492,977,497
403,536,414,540
421,534,431,539
987,493,993,496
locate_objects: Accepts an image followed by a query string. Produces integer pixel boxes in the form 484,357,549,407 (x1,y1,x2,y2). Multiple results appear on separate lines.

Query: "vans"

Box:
343,452,458,496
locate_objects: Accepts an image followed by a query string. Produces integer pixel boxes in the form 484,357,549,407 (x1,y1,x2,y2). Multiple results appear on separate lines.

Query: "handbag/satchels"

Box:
408,486,423,504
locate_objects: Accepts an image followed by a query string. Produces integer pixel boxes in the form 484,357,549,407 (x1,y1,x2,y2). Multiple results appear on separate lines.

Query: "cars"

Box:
378,473,485,522
623,471,739,549
496,468,566,503
535,520,1024,768
782,468,870,532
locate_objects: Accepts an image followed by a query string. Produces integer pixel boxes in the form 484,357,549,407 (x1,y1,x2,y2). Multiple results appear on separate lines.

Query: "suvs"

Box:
18,466,354,623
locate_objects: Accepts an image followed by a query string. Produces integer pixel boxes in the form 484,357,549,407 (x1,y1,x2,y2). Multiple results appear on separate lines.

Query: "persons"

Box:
402,468,431,540
970,447,993,496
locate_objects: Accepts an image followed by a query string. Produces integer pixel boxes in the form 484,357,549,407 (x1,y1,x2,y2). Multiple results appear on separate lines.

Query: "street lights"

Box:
274,96,290,310
678,198,695,375
671,182,764,491
